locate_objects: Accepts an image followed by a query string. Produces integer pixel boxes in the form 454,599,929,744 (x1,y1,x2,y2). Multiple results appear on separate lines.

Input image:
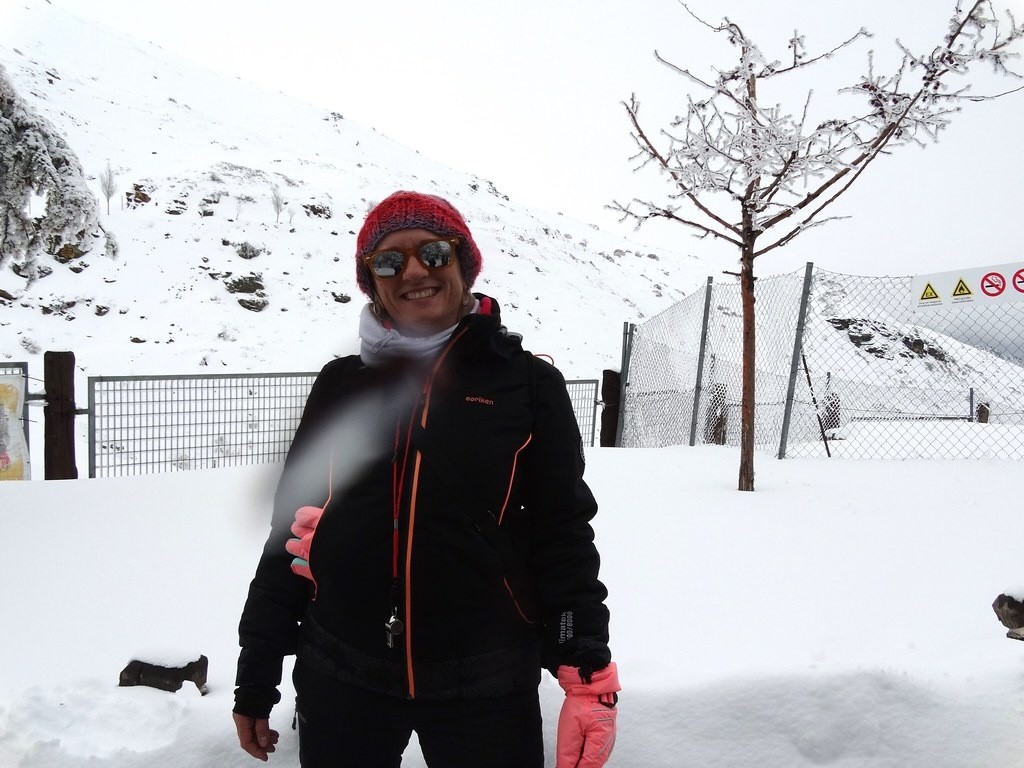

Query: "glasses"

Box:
362,237,460,280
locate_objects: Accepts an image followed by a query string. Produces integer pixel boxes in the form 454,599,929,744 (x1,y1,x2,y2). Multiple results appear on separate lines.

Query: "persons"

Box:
232,191,622,768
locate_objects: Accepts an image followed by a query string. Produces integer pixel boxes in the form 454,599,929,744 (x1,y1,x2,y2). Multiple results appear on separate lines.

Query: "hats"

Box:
355,190,482,293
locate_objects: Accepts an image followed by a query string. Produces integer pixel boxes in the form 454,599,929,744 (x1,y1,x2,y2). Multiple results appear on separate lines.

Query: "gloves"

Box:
557,663,621,768
285,505,322,580
